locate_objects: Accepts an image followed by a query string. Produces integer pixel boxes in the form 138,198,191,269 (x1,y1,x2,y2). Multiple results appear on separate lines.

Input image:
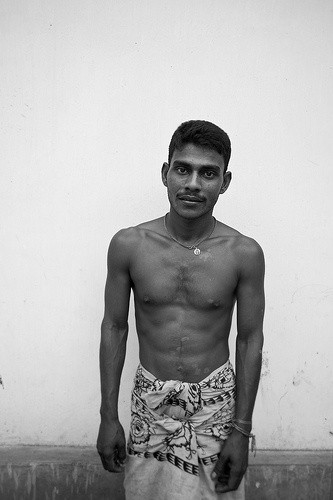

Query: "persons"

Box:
97,120,265,500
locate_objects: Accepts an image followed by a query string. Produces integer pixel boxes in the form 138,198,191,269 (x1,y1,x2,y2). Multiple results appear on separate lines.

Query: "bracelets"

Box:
231,420,256,457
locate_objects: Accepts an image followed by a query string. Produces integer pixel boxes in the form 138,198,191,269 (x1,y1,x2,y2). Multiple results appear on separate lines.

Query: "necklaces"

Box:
164,212,217,256
